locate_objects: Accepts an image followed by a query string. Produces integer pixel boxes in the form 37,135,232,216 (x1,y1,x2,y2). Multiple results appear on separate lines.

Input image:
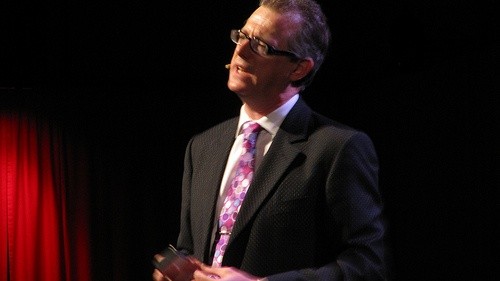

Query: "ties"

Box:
212,121,259,267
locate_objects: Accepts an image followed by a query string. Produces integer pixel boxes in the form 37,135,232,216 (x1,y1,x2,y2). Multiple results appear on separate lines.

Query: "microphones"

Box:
224,64,231,69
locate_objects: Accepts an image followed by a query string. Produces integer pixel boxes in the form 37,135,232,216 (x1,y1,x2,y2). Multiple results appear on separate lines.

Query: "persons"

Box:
154,0,386,281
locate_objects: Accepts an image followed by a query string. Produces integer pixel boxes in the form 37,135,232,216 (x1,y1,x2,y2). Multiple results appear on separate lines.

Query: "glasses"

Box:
230,29,304,65
